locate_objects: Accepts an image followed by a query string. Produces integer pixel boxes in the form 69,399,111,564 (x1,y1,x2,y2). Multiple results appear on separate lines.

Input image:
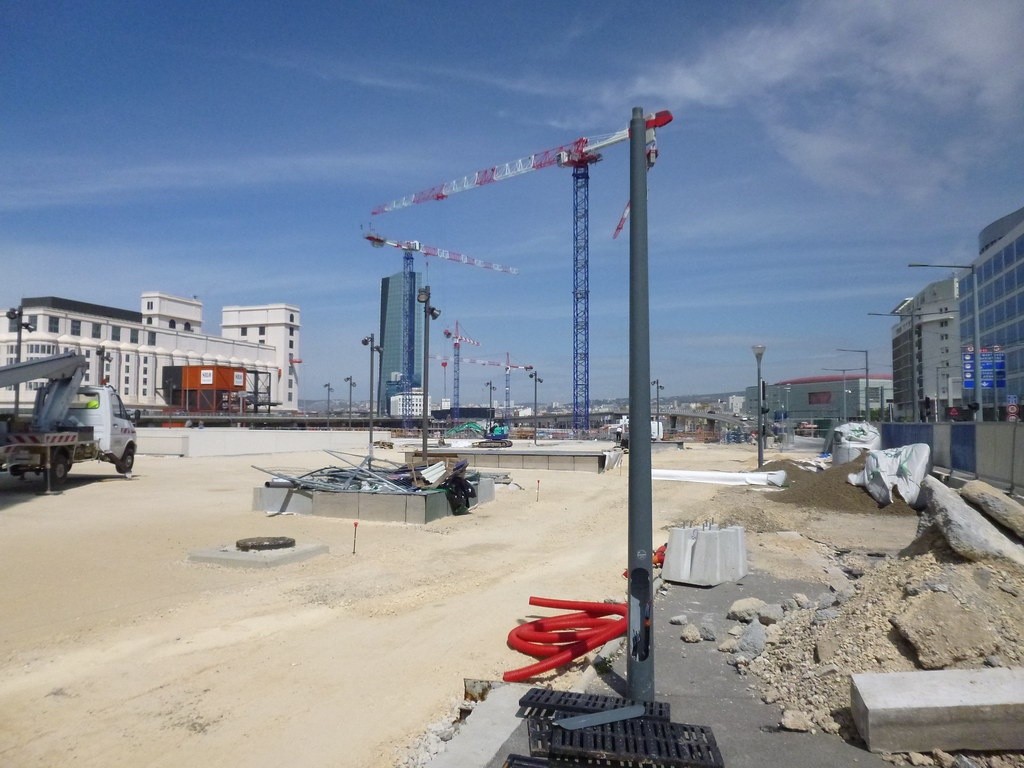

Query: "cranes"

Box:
371,109,672,444
445,317,480,423
365,233,519,431
431,350,534,425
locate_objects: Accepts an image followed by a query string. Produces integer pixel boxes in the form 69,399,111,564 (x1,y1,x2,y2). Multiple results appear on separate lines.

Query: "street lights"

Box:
751,344,767,467
651,378,664,441
345,375,356,429
361,333,385,458
820,366,869,421
838,348,870,421
907,264,983,424
7,305,31,413
867,309,969,422
485,380,497,422
529,370,543,444
417,285,439,459
325,382,334,431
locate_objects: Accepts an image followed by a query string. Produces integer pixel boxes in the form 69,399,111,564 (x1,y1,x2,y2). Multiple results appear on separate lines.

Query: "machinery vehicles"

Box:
0,352,140,495
472,423,513,448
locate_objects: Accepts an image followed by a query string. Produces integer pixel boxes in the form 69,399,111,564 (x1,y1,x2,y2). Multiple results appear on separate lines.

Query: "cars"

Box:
708,411,754,421
